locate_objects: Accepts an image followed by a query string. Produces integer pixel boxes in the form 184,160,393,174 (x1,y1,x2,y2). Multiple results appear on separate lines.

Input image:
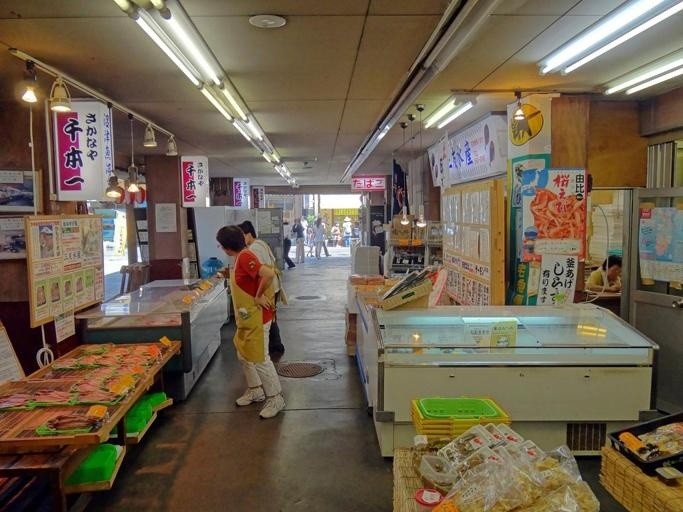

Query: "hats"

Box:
344,217,350,221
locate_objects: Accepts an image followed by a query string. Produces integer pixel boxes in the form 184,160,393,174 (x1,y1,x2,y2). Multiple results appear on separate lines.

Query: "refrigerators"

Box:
73,277,231,402
354,303,659,460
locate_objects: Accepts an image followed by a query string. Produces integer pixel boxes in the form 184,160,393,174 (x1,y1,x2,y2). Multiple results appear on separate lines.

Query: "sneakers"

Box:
235,387,265,406
259,395,286,419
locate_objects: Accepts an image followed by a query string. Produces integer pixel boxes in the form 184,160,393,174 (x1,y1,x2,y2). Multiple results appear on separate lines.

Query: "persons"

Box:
240,220,285,355
586,255,623,292
282,213,353,269
216,227,286,420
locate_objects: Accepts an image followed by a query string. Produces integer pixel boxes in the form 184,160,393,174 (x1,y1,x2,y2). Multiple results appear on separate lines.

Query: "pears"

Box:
510,104,544,146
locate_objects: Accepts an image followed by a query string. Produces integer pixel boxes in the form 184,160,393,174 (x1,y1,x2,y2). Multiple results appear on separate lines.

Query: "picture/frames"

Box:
0,167,43,215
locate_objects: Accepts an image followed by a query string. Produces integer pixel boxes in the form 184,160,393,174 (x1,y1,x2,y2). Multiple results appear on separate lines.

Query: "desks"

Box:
586,292,621,316
0,342,181,511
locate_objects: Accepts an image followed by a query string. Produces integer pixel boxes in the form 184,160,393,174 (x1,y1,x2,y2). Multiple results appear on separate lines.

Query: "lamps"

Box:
423,92,478,129
513,92,526,121
10,48,179,158
114,0,299,188
342,1,504,186
103,102,139,198
537,0,683,97
400,105,427,229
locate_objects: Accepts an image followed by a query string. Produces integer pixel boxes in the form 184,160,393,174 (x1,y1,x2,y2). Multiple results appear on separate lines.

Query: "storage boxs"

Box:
344,275,401,357
